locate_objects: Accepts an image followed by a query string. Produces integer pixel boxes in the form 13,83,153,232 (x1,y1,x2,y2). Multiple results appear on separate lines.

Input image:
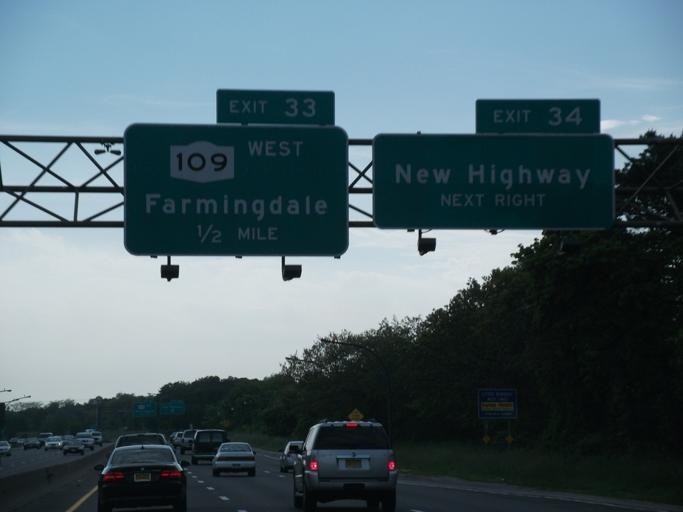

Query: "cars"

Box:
280,418,400,512
0,420,257,512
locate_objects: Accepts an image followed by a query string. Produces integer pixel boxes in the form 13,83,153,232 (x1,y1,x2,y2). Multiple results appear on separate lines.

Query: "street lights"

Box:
0,390,32,408
